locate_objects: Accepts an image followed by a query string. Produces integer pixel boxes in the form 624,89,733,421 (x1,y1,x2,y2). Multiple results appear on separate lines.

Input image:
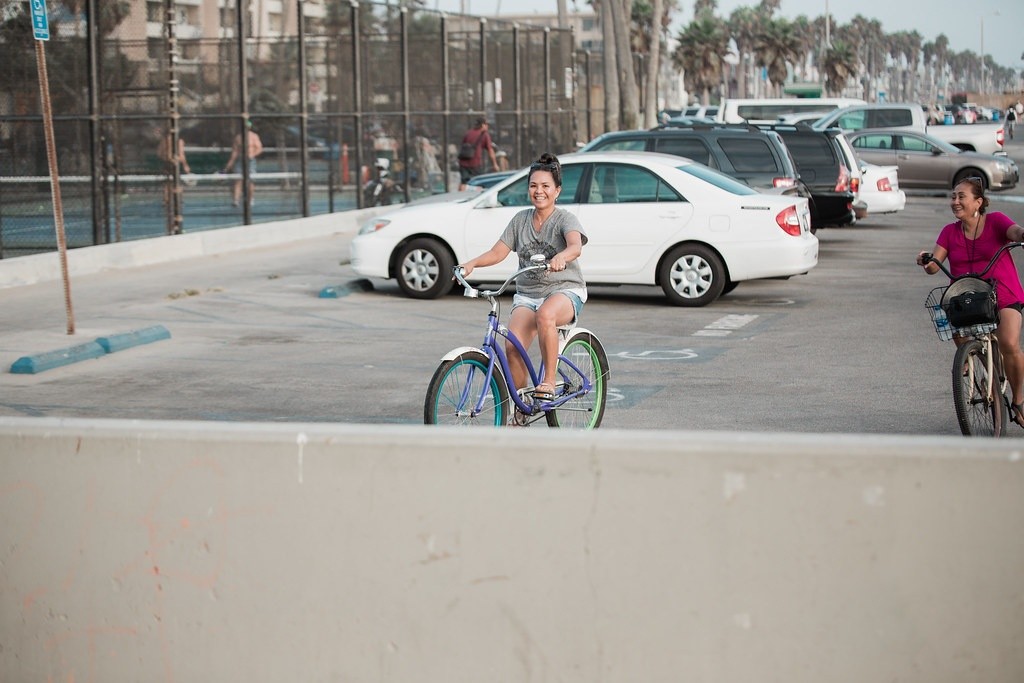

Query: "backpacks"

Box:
1007,109,1015,121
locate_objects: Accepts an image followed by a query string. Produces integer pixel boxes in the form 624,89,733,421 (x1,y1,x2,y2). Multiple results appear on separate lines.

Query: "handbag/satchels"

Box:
947,292,997,329
457,143,475,160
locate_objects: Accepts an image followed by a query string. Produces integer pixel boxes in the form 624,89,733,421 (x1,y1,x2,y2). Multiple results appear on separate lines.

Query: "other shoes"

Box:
233,201,239,206
250,198,255,206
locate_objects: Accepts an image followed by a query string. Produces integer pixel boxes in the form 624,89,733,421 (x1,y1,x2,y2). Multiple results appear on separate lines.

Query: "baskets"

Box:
925,278,1000,341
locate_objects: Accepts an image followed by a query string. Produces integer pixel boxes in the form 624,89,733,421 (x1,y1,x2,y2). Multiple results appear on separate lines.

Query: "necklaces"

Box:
536,213,542,225
963,216,980,274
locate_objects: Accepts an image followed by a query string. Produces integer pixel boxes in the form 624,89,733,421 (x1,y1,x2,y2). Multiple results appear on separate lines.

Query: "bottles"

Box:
933,304,953,341
959,323,997,337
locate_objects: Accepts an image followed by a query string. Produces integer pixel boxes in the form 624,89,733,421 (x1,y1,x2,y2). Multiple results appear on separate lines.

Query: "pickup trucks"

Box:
807,100,1005,156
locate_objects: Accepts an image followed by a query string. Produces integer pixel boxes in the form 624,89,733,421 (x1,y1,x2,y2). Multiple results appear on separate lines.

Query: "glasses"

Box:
958,177,982,192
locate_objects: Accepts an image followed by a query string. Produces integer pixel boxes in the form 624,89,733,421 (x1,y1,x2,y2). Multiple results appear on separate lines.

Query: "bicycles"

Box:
917,232,1024,439
422,253,612,429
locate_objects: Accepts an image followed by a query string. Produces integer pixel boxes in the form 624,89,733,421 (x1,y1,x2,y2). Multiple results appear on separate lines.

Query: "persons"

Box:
157,130,190,206
452,152,588,427
1003,104,1018,140
225,121,262,207
1014,100,1024,124
364,127,446,192
916,176,1024,429
458,117,509,191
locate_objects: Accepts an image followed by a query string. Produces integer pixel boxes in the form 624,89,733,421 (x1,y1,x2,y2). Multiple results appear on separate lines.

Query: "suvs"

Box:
457,120,807,202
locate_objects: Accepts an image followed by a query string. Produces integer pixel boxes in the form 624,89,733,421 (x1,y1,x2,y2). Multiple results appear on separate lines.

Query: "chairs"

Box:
589,178,602,202
602,176,620,203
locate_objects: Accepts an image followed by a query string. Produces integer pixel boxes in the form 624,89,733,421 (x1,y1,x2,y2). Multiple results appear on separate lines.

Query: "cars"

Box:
176,113,341,162
842,127,1020,193
591,96,994,237
342,147,821,308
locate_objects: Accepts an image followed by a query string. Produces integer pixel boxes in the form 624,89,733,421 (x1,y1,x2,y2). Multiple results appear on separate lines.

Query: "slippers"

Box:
963,359,969,377
1012,400,1024,429
535,382,555,395
507,417,528,426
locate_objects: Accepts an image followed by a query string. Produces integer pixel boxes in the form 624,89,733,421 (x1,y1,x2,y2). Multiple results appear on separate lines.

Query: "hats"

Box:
247,121,253,128
939,276,992,312
